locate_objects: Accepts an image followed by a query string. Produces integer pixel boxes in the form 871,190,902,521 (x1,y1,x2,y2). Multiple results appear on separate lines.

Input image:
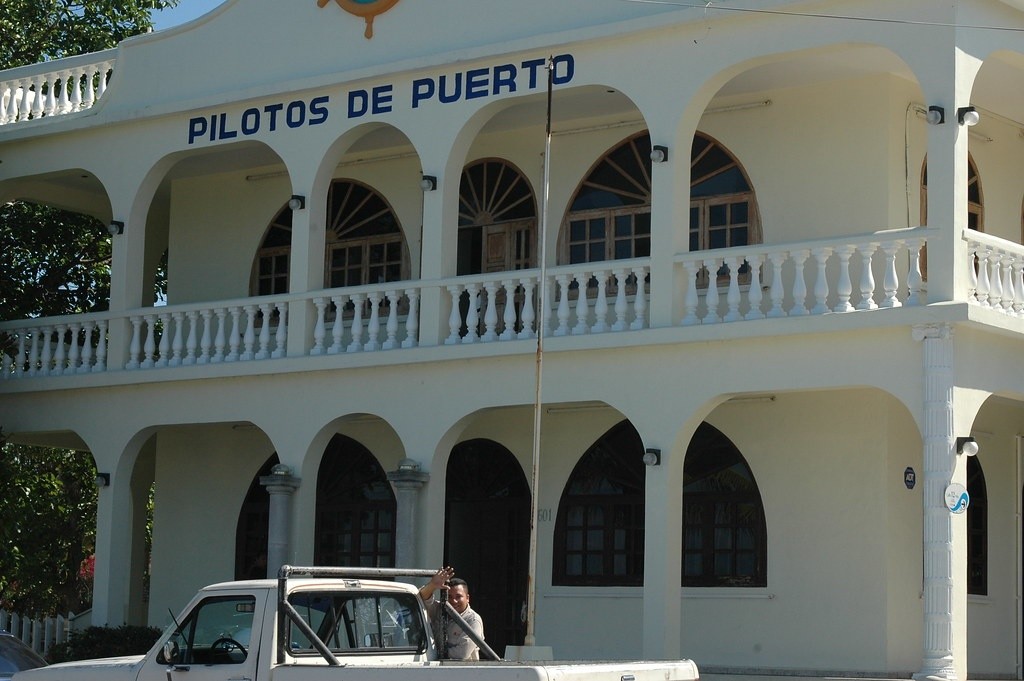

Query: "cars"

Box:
0,631,48,681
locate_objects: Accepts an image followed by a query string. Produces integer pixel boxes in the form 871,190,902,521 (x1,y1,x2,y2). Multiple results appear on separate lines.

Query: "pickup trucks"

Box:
13,565,699,681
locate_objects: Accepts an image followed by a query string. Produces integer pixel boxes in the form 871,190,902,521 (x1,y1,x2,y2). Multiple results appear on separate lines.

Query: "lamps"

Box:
94,473,110,487
419,175,437,192
957,106,979,126
650,145,669,163
926,105,945,126
108,220,124,235
957,437,978,456
289,195,305,211
643,448,662,467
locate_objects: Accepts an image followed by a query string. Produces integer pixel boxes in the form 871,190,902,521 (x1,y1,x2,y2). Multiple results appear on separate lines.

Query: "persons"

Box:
419,566,485,661
